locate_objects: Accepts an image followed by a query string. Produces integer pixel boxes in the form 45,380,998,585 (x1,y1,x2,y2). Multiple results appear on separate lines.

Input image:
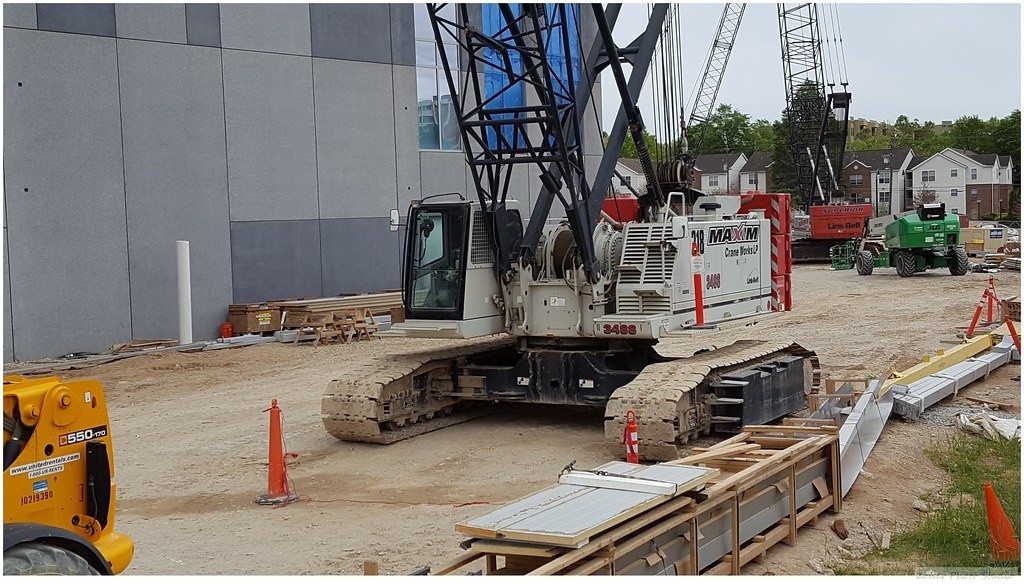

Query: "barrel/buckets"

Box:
220,321,233,339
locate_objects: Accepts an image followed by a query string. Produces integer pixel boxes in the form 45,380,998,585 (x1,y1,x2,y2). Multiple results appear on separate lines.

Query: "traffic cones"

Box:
983,482,1020,562
255,399,298,505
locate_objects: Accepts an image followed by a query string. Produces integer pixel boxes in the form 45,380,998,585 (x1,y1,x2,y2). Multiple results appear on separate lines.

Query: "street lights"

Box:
976,198,982,220
901,170,906,212
876,169,880,218
999,198,1003,220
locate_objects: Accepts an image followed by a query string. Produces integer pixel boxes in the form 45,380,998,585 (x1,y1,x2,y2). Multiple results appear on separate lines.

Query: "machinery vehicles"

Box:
3,374,134,576
856,203,968,276
322,3,821,463
790,92,875,262
